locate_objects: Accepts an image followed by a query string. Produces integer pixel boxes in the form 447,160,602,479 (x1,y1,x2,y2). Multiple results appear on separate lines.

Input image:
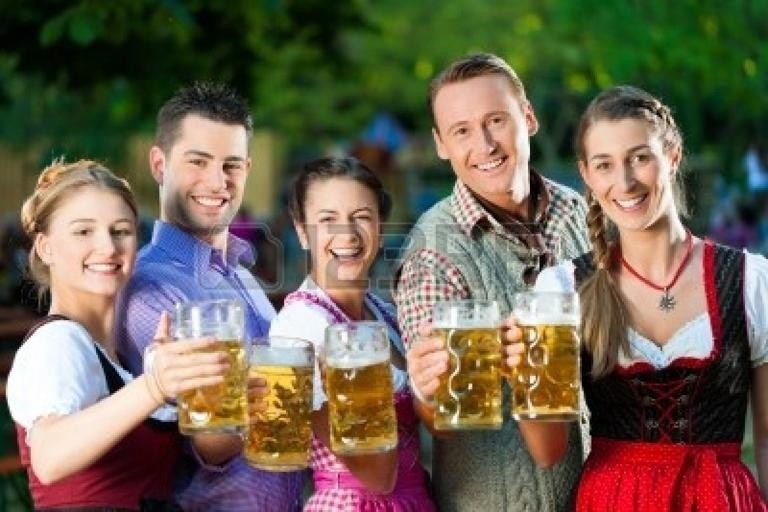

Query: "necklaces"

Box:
616,227,693,312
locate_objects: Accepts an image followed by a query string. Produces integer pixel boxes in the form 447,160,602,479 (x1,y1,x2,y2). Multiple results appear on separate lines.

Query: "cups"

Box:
500,291,579,424
171,299,249,436
321,321,397,458
242,334,316,472
428,300,502,432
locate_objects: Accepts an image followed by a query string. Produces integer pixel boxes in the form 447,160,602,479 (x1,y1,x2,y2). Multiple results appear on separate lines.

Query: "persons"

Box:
5,153,230,512
396,51,609,511
267,156,435,512
112,78,304,512
501,84,768,511
